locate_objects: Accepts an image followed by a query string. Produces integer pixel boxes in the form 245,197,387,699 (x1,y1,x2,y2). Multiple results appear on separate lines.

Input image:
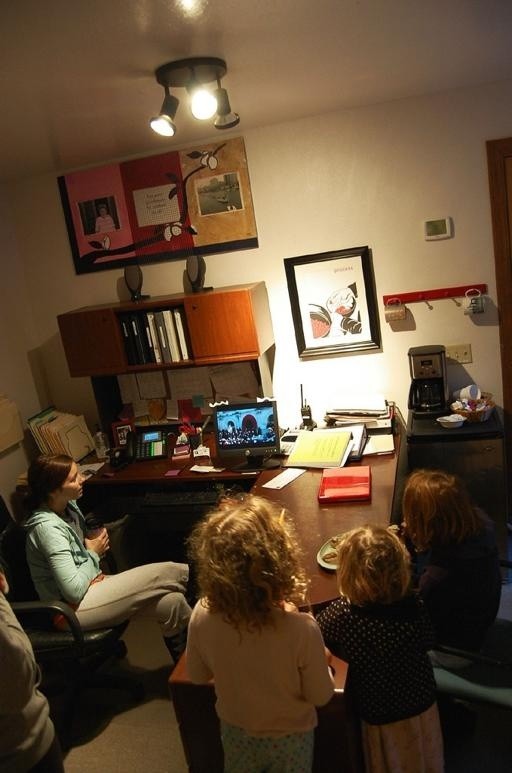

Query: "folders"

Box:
119,314,136,366
128,313,145,364
146,313,162,364
172,307,190,361
312,424,369,462
153,311,171,364
163,308,181,363
284,430,354,470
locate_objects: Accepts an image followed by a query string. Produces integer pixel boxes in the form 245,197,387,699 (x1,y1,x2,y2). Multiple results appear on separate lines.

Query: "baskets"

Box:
450,399,496,423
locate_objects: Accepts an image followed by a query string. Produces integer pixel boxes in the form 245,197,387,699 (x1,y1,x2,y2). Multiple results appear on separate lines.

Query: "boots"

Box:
164,628,187,665
187,564,199,604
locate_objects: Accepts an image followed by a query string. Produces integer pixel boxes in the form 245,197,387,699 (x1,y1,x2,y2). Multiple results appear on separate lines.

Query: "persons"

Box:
9,453,193,667
94,205,116,235
186,504,338,773
1,573,65,773
396,467,501,674
219,422,275,444
313,525,449,773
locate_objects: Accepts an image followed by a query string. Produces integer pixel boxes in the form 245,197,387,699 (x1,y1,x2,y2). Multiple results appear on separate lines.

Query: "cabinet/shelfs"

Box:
406,398,512,569
57,281,276,432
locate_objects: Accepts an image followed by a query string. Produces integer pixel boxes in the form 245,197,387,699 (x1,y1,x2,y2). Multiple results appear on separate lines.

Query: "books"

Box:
26,406,96,462
118,309,189,365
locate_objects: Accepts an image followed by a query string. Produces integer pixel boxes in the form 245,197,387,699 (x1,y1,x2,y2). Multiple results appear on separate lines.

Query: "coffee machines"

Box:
408,345,451,421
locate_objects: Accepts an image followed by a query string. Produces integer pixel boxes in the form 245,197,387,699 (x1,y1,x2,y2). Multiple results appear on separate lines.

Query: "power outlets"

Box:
445,343,472,364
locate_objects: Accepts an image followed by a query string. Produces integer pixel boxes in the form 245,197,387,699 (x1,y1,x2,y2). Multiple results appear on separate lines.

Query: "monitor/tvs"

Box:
214,400,281,471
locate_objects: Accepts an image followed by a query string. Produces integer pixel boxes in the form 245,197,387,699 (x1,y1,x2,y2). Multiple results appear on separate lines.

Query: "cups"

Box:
86,518,108,561
453,386,482,401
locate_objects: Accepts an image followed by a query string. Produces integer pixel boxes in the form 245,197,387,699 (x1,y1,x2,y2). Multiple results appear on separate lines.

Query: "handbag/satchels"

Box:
465,288,485,315
384,298,406,323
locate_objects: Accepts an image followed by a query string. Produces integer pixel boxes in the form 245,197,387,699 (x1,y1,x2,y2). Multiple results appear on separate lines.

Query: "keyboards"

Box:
142,491,221,505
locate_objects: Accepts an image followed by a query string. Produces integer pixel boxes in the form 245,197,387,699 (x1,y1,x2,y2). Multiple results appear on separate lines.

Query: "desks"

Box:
248,422,401,608
14,432,261,534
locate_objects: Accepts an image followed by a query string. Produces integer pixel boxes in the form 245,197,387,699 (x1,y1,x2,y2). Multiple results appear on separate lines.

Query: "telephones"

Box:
126,429,168,463
299,383,317,431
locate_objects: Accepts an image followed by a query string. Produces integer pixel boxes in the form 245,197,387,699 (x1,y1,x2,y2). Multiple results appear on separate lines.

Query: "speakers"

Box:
124,265,144,305
186,256,202,293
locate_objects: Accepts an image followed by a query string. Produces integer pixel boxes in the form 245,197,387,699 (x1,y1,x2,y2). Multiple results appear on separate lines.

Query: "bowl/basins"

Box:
436,415,469,429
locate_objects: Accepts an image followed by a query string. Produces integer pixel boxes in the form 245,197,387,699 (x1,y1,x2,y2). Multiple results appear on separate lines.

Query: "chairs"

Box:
0,524,131,663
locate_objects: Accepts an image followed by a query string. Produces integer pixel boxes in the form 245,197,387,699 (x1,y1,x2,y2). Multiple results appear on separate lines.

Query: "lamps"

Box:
149,56,240,136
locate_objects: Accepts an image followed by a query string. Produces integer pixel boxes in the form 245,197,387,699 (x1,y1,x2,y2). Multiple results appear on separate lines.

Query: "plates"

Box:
317,537,339,571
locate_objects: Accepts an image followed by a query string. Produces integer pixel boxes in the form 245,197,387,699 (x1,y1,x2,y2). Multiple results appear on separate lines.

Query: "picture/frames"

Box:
56,136,259,276
284,246,380,358
112,419,136,447
135,370,171,400
